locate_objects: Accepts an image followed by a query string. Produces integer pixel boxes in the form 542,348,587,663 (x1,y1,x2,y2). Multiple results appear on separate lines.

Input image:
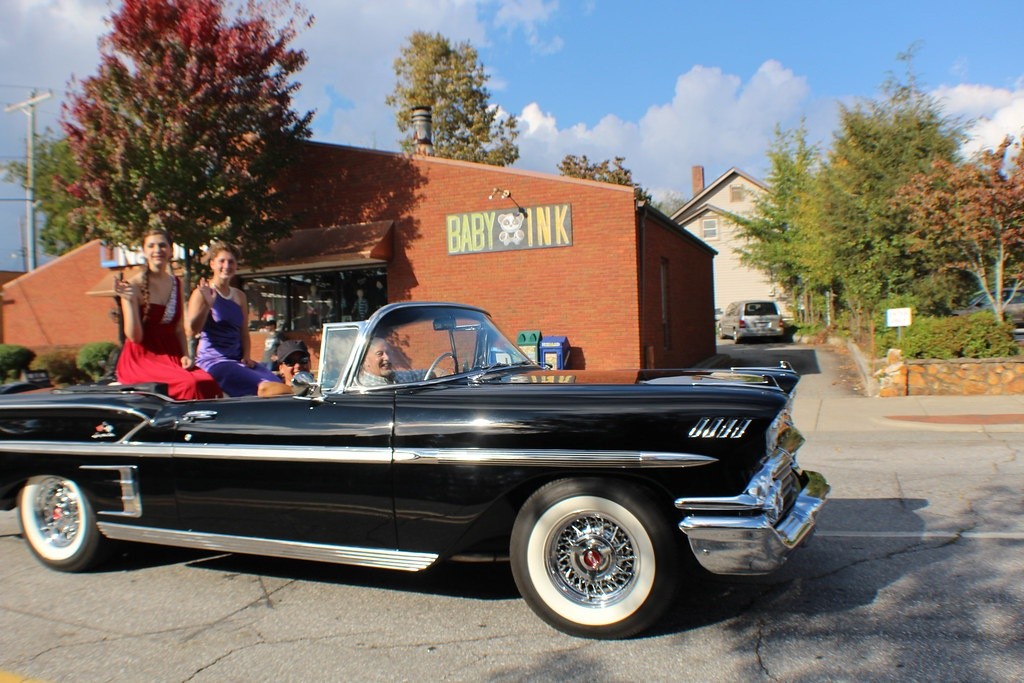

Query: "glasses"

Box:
284,355,309,366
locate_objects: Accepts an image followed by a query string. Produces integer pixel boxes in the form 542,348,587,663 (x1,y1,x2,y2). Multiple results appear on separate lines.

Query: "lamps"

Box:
488,187,527,217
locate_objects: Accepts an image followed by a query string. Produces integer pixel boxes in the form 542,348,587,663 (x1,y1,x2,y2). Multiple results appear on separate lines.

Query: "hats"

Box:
277,340,309,362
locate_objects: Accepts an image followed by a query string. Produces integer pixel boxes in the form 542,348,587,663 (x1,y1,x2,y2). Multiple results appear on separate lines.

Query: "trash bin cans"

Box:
538,335,571,371
516,330,541,367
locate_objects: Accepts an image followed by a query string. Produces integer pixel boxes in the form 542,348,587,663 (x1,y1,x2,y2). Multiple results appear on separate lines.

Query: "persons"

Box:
352,288,370,322
350,333,439,394
374,280,387,311
324,297,336,325
260,319,285,373
259,299,284,331
183,239,288,399
276,336,312,396
108,228,224,402
307,284,324,333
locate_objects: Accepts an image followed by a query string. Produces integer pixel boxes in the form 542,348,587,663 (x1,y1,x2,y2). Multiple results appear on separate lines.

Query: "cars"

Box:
952,287,1024,328
719,302,785,343
0,300,825,640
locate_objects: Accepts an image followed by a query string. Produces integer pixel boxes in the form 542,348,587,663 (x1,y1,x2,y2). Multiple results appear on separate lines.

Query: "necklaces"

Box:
210,281,235,301
